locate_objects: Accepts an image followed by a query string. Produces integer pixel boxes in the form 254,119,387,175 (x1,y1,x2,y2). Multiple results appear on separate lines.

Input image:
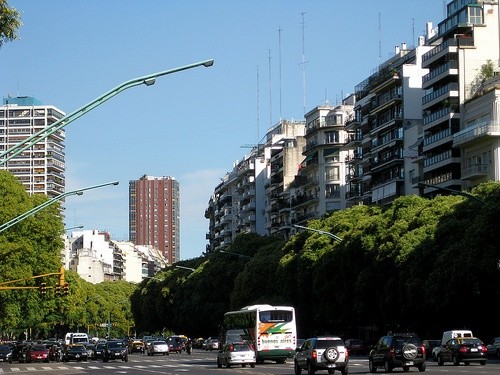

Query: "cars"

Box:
0,332,129,364
436,337,487,365
130,335,223,355
296,339,306,350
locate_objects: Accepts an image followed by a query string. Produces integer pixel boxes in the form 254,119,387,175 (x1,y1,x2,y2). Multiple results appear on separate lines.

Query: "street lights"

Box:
108,301,124,340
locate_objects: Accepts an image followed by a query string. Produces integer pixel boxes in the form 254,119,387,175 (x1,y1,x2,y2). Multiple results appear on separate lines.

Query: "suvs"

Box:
344,338,368,357
216,341,256,367
293,336,349,375
147,341,169,356
422,340,441,357
368,331,426,373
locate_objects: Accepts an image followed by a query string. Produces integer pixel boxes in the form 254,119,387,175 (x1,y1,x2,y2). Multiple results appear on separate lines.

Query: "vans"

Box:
486,337,500,359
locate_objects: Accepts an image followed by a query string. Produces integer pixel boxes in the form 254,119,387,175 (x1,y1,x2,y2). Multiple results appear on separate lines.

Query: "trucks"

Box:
432,329,473,361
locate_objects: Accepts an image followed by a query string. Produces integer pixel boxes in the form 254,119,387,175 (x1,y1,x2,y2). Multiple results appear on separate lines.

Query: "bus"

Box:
223,304,297,364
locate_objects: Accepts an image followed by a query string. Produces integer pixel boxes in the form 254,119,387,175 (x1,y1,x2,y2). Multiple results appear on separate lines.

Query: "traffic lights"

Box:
63,282,69,297
55,284,62,298
40,283,47,296
